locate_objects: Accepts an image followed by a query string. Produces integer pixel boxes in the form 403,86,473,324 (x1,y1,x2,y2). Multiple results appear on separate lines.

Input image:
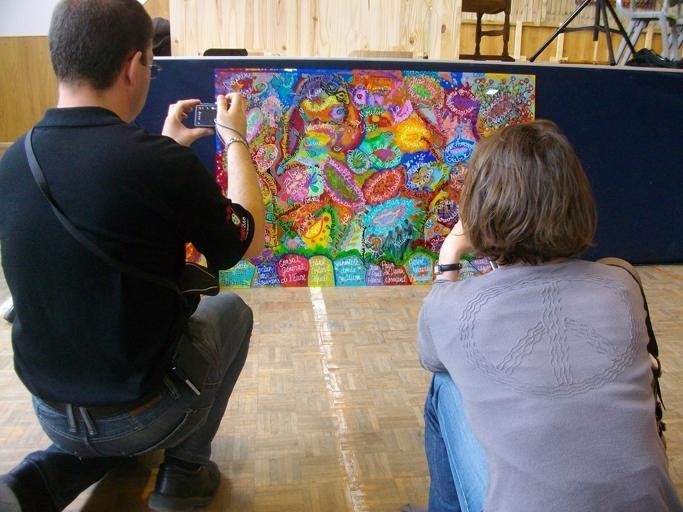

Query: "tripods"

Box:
528,0,640,66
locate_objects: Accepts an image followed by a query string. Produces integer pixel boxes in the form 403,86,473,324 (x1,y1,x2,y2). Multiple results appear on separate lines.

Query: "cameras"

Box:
193,103,219,128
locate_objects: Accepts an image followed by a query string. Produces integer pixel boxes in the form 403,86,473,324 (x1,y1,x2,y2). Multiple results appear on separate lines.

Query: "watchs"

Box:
433,264,462,274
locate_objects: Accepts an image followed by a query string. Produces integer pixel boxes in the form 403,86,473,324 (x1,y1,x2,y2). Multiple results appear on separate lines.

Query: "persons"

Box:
402,119,683,512
0,0,264,512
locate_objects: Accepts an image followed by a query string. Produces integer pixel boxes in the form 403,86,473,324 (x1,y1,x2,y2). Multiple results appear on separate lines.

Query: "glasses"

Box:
144,62,162,80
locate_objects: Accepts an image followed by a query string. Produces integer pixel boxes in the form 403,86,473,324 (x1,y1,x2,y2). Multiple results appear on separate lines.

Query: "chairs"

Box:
614,0,683,67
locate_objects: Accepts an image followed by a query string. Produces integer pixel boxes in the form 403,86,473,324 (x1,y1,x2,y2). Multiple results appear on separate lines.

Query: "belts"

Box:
42,393,168,420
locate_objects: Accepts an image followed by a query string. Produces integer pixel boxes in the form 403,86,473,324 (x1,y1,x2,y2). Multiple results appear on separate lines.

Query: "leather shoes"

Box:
0,460,60,512
147,460,219,511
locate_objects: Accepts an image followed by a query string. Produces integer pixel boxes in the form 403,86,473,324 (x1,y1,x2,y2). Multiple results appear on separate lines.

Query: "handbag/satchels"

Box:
160,263,219,321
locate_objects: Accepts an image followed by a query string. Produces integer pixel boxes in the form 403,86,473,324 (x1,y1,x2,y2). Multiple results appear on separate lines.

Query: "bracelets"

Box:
225,137,249,151
